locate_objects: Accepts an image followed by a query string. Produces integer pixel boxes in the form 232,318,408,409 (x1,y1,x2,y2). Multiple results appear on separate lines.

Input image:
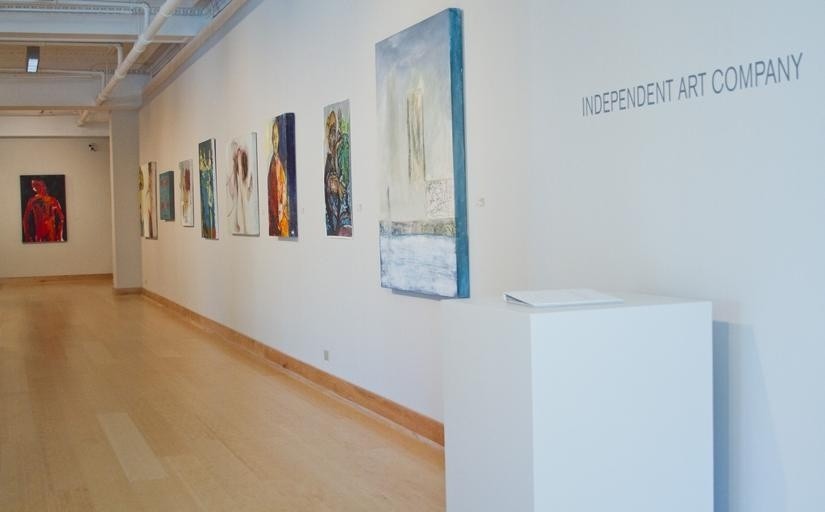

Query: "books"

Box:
501,285,624,307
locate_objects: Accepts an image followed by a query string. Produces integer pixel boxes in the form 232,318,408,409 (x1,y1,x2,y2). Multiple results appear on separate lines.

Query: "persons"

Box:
266,120,291,240
222,144,253,238
22,176,67,241
